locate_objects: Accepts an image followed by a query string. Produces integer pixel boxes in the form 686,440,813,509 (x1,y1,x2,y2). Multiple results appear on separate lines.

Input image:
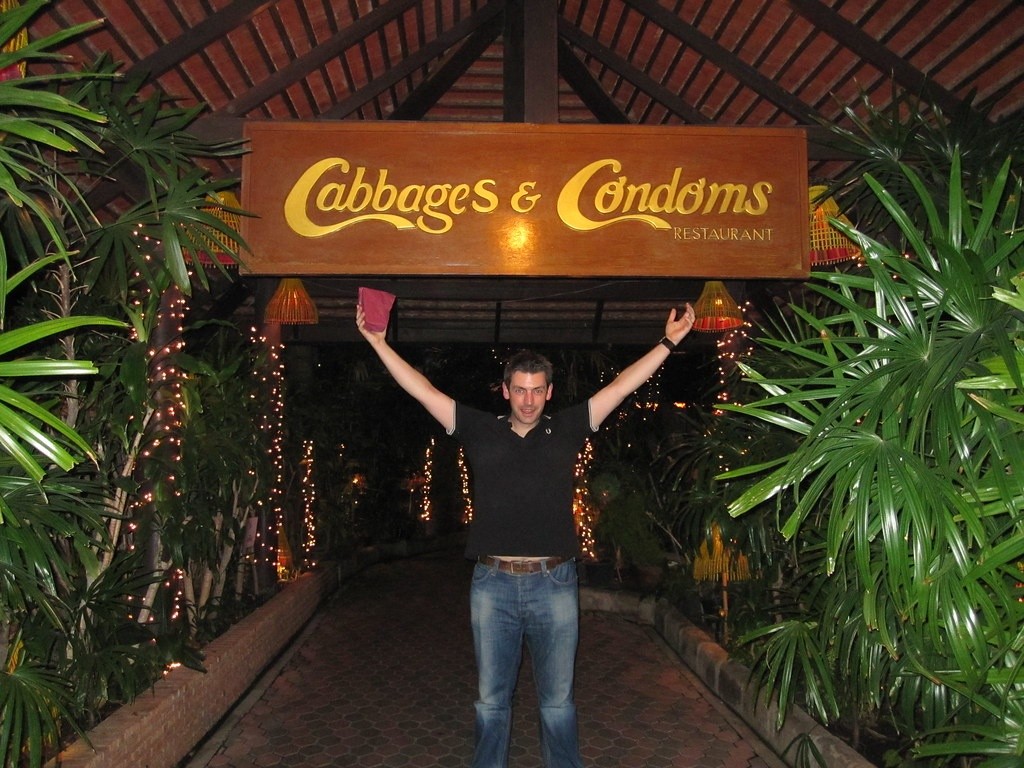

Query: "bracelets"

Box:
659,336,676,353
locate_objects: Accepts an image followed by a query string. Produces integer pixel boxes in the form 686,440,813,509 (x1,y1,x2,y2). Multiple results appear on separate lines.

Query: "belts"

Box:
479,555,572,575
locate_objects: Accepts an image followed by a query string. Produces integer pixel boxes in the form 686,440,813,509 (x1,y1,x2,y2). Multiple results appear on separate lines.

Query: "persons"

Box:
355,301,696,768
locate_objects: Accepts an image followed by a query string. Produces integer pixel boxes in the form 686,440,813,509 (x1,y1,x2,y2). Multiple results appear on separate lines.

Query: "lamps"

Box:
181,191,242,269
692,281,744,333
263,278,318,325
809,185,862,264
